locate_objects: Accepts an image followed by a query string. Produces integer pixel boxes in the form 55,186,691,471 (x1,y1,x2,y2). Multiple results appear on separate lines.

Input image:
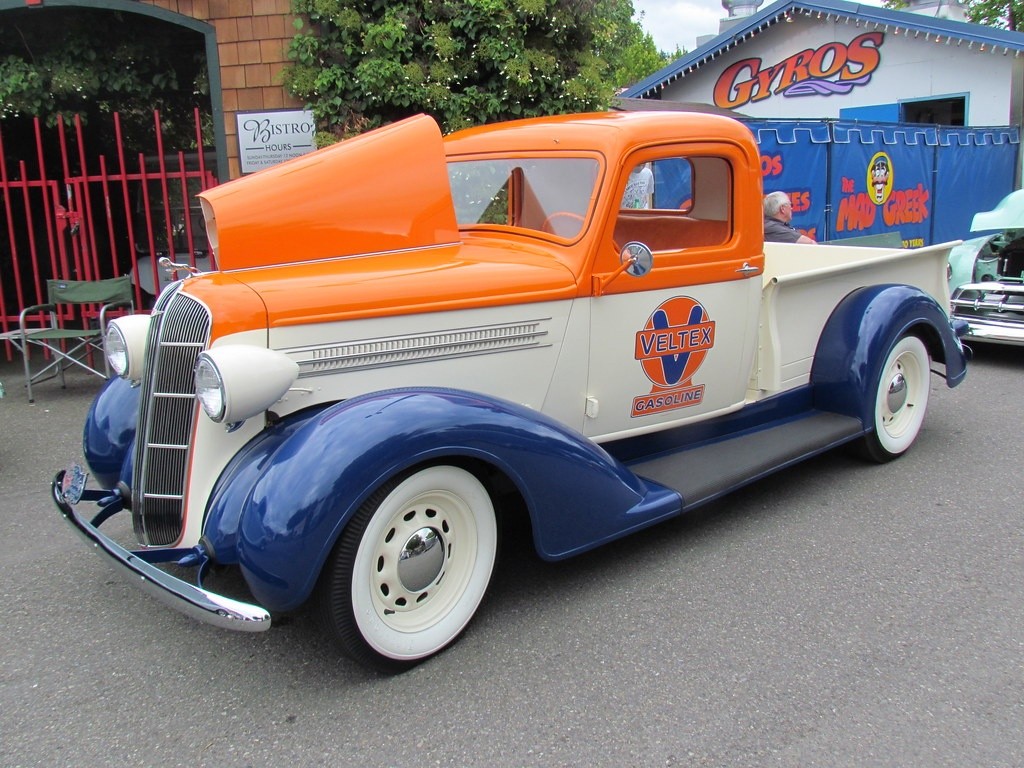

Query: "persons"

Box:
763,191,817,245
619,160,656,211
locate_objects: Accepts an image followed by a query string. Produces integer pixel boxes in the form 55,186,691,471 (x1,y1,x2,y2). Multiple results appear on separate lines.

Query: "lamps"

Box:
786,11,796,23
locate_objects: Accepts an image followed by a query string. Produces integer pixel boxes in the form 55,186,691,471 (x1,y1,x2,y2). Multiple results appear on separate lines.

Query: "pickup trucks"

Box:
50,108,974,677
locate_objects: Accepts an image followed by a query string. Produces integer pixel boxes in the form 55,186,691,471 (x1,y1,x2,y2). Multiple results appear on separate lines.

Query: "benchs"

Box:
613,214,727,253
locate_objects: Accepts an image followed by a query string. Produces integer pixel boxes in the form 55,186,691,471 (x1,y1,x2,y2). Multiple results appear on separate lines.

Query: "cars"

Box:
950,187,1024,349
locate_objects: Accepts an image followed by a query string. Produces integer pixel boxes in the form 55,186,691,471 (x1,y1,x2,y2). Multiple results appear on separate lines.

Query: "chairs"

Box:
997,239,1024,282
0,274,136,404
